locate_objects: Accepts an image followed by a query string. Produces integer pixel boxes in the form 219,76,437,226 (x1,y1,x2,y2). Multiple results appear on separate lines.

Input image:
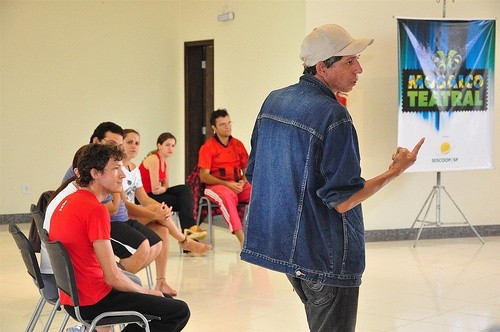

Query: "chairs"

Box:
8,164,248,332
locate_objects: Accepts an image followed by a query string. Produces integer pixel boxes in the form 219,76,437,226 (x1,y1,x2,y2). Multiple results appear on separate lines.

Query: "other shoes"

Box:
185,231,208,241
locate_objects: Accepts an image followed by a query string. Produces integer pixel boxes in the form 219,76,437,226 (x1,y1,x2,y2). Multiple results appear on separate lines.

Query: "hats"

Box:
299,23,375,69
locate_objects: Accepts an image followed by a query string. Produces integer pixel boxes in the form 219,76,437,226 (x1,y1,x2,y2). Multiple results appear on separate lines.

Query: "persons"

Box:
47,143,194,332
38,122,213,332
136,132,212,254
239,23,424,332
198,110,253,254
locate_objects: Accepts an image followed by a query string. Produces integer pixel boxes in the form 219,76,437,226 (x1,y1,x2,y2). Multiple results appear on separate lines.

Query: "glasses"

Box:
216,120,232,128
102,136,124,149
